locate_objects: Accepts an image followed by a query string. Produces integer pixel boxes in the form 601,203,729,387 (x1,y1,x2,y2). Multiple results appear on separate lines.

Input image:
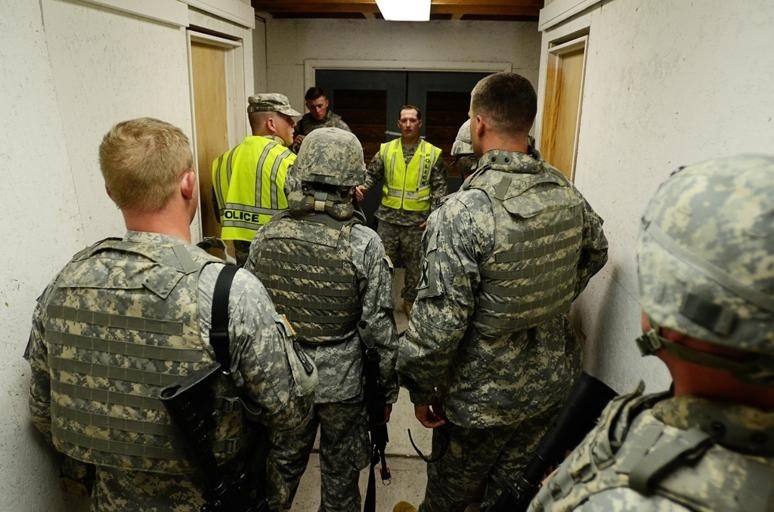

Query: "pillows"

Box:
294,127,365,186
638,154,774,353
449,119,532,156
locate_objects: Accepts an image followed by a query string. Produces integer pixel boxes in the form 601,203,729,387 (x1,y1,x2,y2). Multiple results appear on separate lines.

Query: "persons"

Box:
421,119,536,264
208,93,363,271
527,155,774,512
25,118,319,512
294,88,351,153
241,127,399,512
392,71,605,512
358,104,447,322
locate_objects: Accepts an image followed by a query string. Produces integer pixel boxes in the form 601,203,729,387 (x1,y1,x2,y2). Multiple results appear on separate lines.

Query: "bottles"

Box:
247,93,302,116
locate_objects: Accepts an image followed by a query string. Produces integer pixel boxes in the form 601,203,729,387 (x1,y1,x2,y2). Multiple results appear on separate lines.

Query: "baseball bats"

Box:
488,371,618,512
158,364,271,511
355,319,394,486
